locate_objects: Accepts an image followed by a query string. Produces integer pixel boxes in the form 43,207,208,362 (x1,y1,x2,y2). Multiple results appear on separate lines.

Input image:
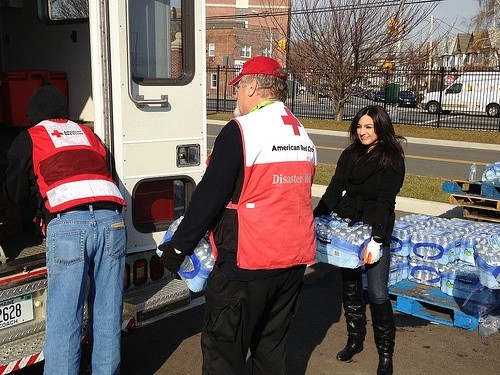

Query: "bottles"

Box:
361,214,500,305
482,162,500,185
157,216,216,292
313,212,372,246
469,163,477,182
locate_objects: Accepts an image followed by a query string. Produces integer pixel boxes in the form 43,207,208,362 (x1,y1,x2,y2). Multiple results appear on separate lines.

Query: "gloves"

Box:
157,241,186,274
363,236,383,265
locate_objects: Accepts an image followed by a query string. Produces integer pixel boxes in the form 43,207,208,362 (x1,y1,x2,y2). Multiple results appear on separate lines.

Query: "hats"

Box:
227,56,287,86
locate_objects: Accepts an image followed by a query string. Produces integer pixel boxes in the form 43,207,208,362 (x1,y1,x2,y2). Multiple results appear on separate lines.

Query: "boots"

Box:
369,299,396,375
336,281,367,361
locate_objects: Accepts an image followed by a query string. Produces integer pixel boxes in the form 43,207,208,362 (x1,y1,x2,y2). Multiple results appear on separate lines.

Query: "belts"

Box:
60,205,122,213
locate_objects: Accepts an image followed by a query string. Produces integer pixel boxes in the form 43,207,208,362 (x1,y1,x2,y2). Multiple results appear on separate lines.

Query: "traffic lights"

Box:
278,39,283,51
283,40,288,51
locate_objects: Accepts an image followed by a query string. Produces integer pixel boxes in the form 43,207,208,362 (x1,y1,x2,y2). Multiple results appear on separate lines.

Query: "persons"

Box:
0,85,128,375
157,56,316,375
312,104,406,375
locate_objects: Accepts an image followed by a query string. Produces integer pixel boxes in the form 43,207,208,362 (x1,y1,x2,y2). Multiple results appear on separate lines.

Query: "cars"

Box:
298,84,418,107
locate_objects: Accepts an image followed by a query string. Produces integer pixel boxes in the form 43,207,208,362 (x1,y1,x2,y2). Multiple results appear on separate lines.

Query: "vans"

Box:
421,71,500,118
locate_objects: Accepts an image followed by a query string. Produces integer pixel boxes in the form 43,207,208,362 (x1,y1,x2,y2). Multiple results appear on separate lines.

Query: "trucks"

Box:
0,0,220,375
